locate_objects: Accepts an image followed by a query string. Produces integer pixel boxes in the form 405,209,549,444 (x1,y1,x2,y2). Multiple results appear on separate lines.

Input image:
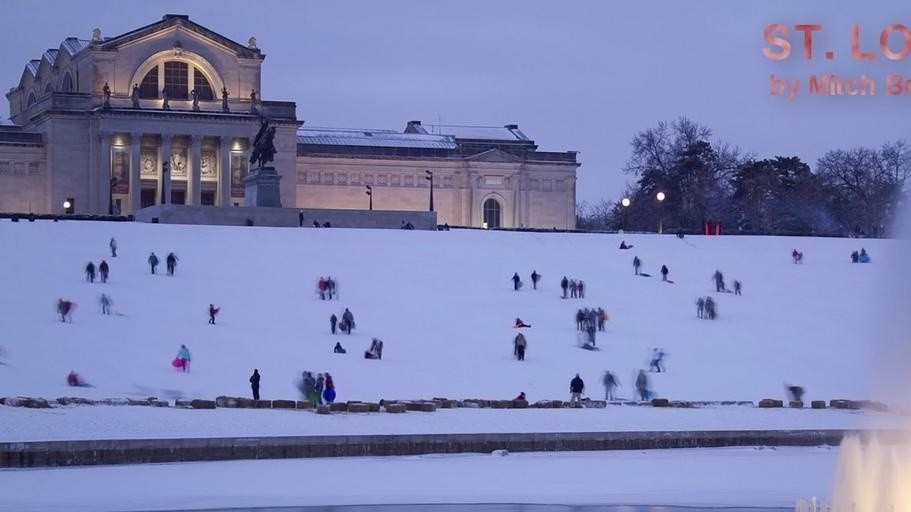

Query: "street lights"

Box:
65,202,71,214
622,197,630,231
110,177,117,214
161,161,168,204
426,171,433,210
366,185,372,210
657,193,665,233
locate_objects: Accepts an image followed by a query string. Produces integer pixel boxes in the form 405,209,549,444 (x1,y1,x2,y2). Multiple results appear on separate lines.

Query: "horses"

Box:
256,126,277,167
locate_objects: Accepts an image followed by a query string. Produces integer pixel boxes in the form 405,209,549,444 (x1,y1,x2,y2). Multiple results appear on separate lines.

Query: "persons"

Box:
792,248,800,264
510,226,744,400
253,113,277,154
55,208,383,404
249,88,259,111
189,85,201,108
130,82,143,109
400,219,415,230
860,248,866,256
220,85,229,110
161,86,172,108
102,82,113,104
850,250,859,263
